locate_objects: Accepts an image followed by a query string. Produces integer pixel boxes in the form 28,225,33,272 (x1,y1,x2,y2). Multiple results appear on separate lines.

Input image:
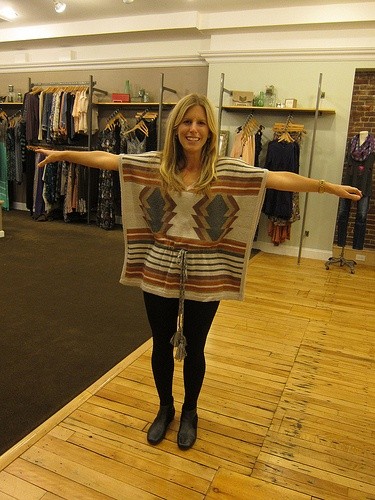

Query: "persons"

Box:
36,94,363,451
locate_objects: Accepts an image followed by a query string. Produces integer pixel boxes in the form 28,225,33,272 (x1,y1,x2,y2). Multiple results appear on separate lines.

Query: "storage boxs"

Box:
232,91,253,106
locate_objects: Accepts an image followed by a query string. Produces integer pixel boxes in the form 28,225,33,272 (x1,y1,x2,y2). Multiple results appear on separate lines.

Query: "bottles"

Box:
256,91,265,106
7,84,22,103
124,80,134,98
138,88,149,102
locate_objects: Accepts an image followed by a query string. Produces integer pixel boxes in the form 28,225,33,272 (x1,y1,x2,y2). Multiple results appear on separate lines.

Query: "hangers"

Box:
29,82,90,93
235,114,265,136
0,107,22,127
273,115,304,143
122,108,159,137
104,109,127,132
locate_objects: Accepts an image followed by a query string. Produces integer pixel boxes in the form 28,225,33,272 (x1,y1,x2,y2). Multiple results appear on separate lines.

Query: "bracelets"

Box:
319,180,324,193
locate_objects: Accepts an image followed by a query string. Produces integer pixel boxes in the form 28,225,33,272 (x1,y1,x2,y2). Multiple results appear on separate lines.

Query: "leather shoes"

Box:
147,403,175,445
177,407,198,450
